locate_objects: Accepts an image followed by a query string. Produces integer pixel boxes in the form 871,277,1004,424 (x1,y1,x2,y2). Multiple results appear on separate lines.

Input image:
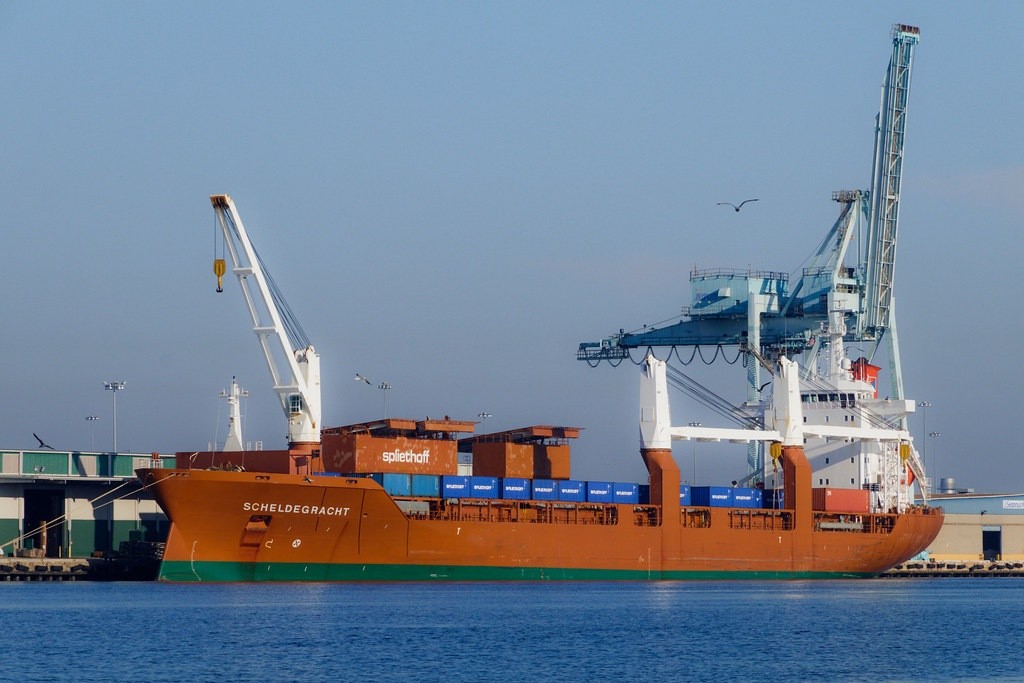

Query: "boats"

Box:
133,191,951,585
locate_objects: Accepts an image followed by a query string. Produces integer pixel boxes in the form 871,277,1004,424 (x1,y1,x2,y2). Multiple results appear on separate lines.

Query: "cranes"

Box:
562,22,923,486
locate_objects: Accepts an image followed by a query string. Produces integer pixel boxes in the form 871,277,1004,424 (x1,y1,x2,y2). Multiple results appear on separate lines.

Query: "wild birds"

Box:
33,432,55,451
717,199,759,212
355,374,372,385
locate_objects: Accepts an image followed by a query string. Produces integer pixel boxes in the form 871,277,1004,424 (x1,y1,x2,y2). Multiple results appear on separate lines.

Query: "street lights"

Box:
917,400,932,467
102,380,128,453
477,412,494,435
84,415,100,452
377,381,392,420
928,431,941,494
688,422,703,487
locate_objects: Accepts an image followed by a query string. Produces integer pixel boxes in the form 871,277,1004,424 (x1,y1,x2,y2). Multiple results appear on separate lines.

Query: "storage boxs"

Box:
318,472,870,514
